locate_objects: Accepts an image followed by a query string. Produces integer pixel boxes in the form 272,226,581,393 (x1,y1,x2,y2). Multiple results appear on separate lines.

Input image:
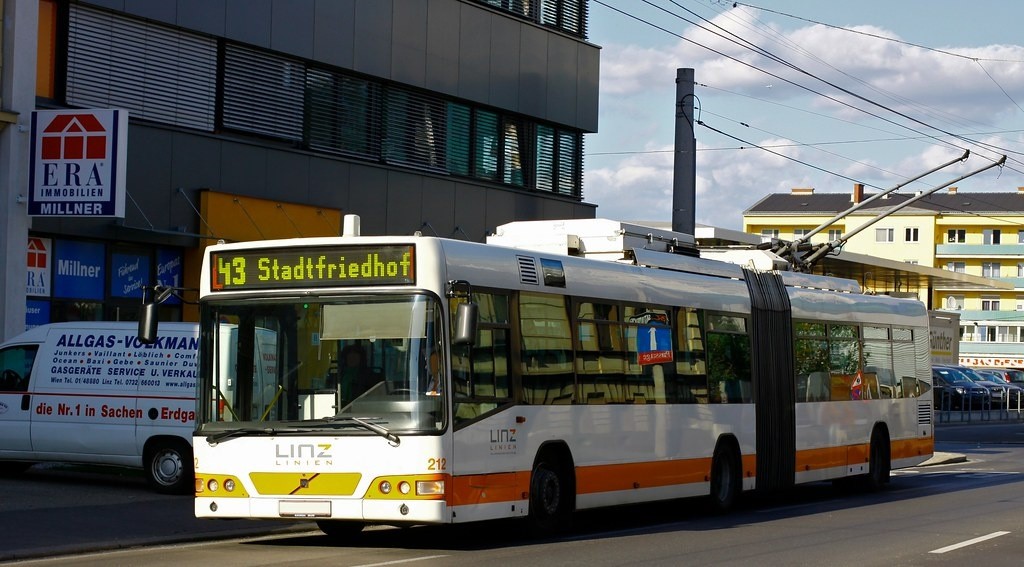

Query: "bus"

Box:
138,214,933,537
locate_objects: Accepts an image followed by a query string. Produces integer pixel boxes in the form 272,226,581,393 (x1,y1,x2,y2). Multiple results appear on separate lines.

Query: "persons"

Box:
424,352,443,396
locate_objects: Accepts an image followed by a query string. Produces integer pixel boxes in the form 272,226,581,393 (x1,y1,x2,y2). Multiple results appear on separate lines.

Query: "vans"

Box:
0,321,288,495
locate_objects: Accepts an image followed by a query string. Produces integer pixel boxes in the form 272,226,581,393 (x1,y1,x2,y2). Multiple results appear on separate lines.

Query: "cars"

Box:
931,366,991,410
974,369,1024,389
953,367,1006,410
978,372,1024,409
